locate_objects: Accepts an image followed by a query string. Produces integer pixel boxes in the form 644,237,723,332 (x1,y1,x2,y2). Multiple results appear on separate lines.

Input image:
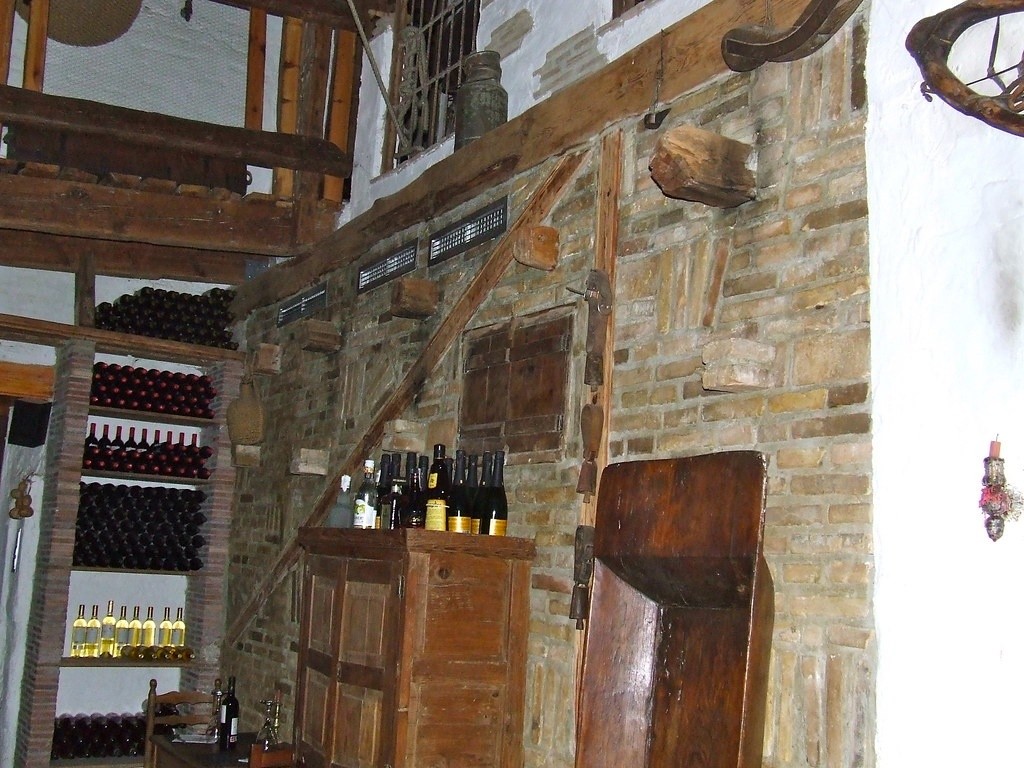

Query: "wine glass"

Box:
255,700,284,752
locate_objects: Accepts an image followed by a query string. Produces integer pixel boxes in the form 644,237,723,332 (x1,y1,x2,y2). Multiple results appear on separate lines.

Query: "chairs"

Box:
143,678,222,768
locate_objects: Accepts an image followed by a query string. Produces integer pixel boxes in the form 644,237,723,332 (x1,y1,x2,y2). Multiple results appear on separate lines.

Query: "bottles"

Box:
50,699,186,761
84,605,101,657
219,676,239,748
100,600,117,658
94,287,239,350
453,50,508,152
113,606,129,658
70,604,87,658
326,443,508,536
71,362,219,572
142,607,156,645
158,607,172,646
121,644,196,663
128,606,142,646
171,608,185,647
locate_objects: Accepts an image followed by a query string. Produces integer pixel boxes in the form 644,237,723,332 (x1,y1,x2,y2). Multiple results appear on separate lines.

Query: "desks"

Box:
150,731,259,768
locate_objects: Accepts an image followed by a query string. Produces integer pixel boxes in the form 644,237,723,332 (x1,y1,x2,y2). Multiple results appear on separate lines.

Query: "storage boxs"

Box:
249,741,293,768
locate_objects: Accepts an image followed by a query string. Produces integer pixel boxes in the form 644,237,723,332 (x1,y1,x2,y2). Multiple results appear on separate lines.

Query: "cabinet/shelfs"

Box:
18,327,238,768
289,524,537,768
